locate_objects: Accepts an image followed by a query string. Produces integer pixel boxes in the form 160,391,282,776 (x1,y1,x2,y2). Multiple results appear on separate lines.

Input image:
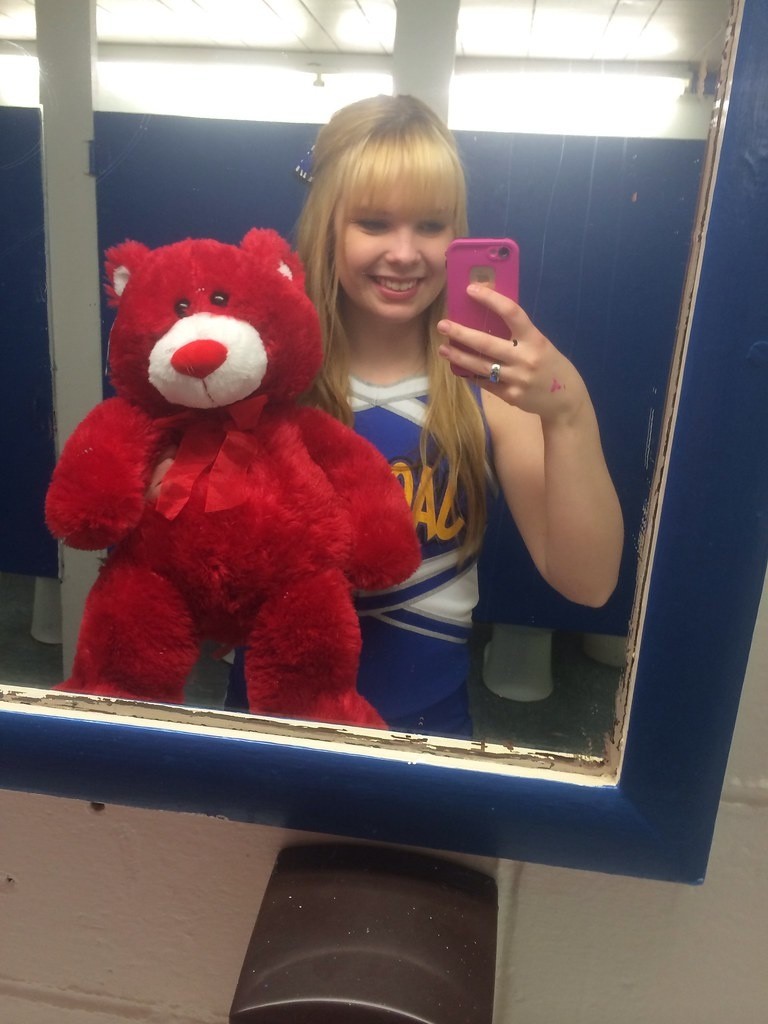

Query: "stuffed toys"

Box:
43,227,420,730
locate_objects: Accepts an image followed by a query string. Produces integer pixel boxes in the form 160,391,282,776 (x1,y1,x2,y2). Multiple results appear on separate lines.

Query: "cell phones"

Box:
445,238,520,377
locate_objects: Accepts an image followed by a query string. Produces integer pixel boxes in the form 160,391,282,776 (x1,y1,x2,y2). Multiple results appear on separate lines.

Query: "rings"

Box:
490,363,500,383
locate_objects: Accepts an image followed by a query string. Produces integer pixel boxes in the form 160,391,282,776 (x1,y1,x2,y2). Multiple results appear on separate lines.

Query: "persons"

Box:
145,94,624,741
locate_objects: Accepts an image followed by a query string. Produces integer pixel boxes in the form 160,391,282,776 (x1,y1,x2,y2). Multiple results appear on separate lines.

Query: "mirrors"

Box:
0,0,768,888
